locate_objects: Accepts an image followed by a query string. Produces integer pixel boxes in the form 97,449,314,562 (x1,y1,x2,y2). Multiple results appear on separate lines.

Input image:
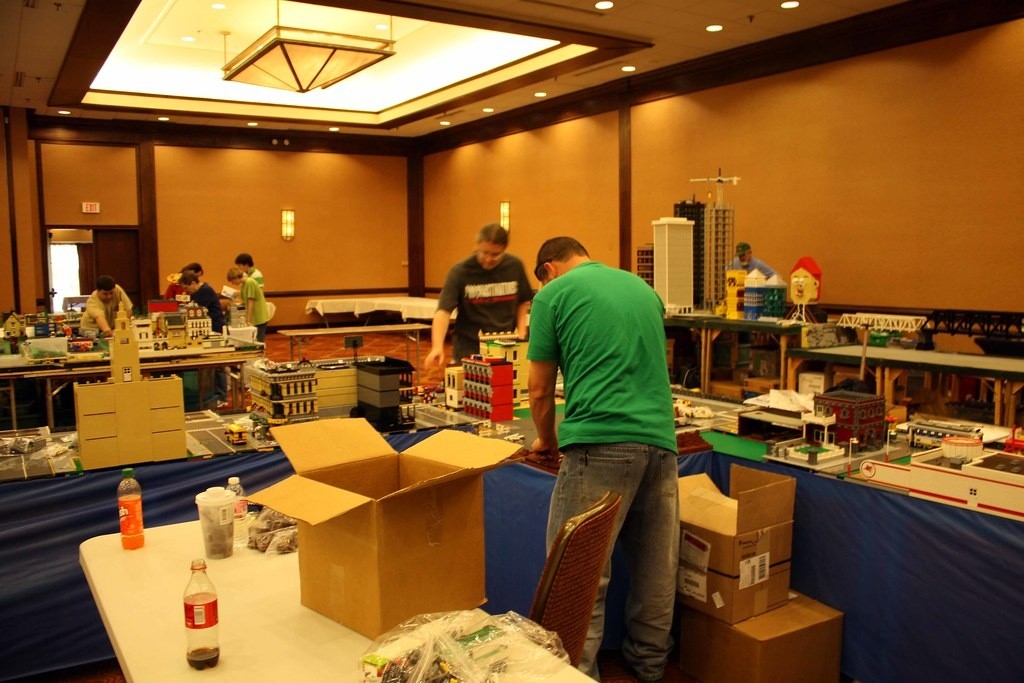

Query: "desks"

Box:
0,294,1024,683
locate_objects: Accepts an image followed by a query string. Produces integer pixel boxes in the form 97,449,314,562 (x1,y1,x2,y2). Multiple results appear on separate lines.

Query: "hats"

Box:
735,242,751,255
228,268,243,281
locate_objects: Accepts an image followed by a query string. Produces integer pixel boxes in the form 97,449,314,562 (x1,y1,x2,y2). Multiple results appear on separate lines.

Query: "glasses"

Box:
534,258,552,281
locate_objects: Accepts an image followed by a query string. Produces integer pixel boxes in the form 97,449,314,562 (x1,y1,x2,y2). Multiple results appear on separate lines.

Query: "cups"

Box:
195,487,236,558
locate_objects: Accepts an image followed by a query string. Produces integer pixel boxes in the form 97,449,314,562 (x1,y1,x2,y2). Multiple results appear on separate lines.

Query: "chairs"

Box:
147,300,179,313
63,296,91,312
527,490,626,672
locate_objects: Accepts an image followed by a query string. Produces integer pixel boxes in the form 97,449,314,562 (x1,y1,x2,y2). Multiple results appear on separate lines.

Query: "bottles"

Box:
225,477,249,547
183,559,220,670
117,468,144,549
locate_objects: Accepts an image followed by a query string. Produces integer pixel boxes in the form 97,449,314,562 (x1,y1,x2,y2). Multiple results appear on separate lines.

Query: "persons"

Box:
178,270,229,408
235,253,264,294
724,243,777,277
164,263,203,304
37,299,48,313
423,224,535,371
227,268,271,343
526,237,680,682
78,275,134,339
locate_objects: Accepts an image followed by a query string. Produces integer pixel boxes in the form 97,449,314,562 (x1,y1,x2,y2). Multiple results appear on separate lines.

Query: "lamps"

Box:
220,0,397,96
499,200,511,235
281,209,296,242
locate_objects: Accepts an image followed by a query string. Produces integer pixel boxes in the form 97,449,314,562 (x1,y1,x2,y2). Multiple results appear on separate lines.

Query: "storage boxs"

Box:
676,464,796,628
749,344,781,376
228,325,258,341
798,371,834,398
25,336,68,359
744,377,782,394
711,380,745,401
241,414,526,647
679,588,844,683
